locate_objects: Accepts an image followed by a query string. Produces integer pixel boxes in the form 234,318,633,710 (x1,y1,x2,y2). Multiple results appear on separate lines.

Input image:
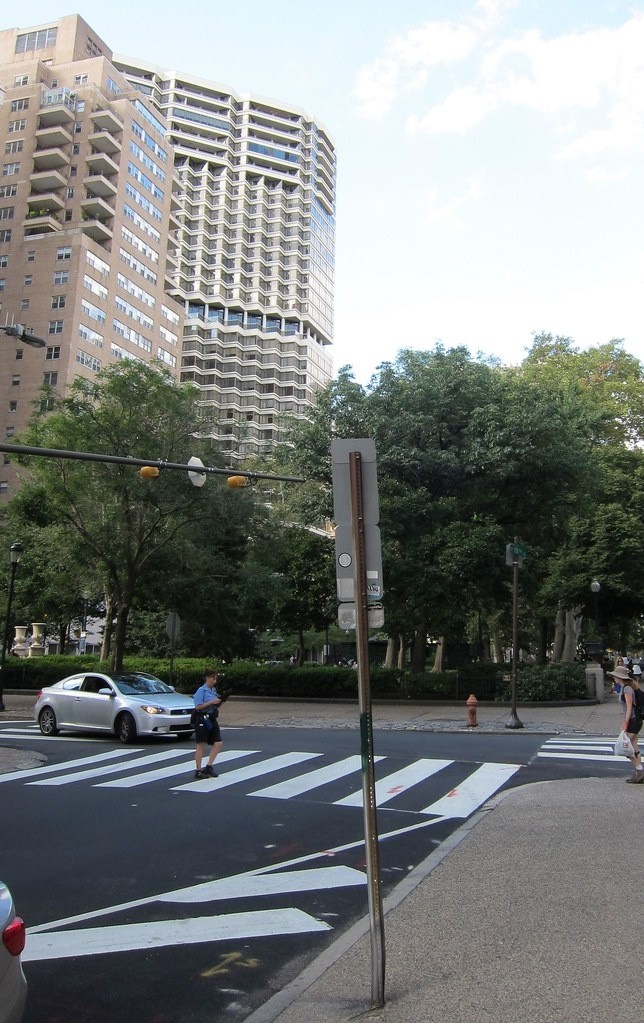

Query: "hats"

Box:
608,667,632,680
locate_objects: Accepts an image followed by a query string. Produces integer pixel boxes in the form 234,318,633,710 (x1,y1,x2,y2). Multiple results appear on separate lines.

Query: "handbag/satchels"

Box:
614,731,635,758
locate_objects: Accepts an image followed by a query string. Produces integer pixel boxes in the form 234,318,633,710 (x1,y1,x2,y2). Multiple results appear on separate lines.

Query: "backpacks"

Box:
621,685,644,720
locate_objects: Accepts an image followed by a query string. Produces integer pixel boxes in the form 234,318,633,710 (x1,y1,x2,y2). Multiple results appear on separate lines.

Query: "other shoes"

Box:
203,765,218,777
195,770,210,779
627,769,644,783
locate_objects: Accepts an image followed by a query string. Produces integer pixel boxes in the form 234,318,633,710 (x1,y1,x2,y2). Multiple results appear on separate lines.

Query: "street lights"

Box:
80,589,92,655
590,578,600,622
2,542,25,662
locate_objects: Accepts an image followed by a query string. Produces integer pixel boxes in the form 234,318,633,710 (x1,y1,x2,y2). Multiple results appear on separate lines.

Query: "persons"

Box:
351,661,358,669
193,670,223,779
290,655,296,665
607,655,644,783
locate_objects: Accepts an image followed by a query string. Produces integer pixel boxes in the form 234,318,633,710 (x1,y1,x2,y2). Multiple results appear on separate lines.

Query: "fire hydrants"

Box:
466,694,480,727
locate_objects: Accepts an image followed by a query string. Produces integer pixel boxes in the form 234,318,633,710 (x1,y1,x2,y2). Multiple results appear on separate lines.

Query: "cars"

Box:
264,661,284,666
34,670,197,744
